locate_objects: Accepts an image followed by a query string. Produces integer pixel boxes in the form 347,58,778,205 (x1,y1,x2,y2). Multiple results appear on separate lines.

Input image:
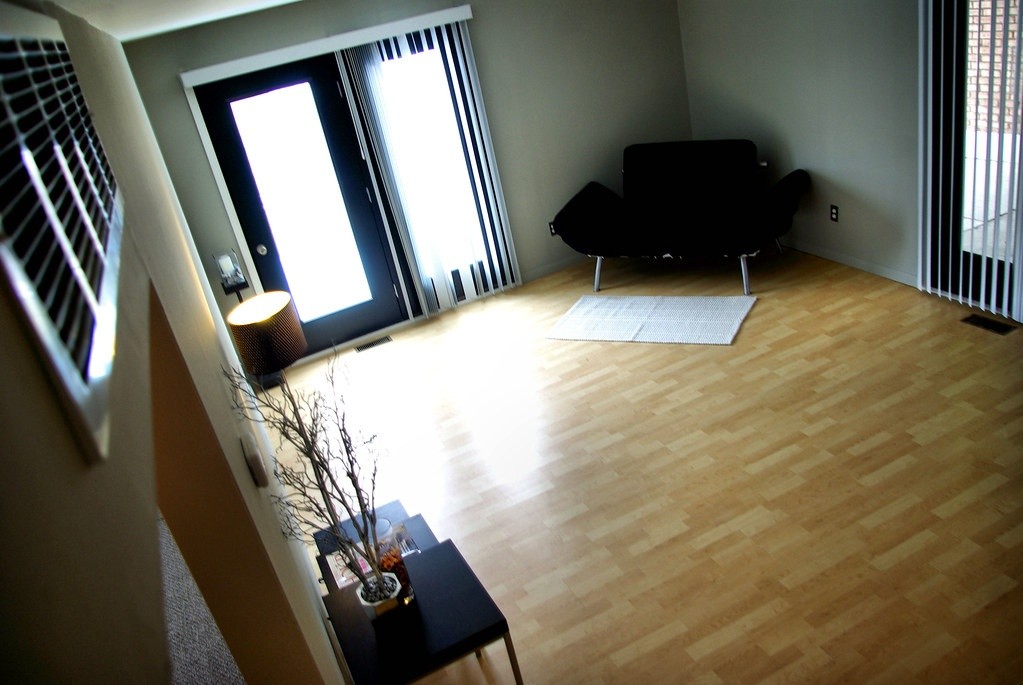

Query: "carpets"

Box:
546,294,756,345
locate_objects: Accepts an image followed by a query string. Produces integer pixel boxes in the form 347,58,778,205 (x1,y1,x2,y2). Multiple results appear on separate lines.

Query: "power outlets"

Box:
831,205,838,221
549,223,557,235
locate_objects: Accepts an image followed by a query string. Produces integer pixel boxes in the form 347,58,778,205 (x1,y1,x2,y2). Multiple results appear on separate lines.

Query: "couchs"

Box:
551,141,813,296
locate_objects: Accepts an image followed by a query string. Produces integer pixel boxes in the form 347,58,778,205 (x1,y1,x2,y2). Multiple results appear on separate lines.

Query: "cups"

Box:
376,517,393,542
377,539,414,605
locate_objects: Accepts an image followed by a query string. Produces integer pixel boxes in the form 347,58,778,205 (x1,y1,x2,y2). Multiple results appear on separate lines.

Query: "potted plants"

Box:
222,335,401,623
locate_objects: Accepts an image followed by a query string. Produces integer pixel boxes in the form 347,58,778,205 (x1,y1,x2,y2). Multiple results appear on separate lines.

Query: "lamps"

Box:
213,247,285,390
225,290,338,526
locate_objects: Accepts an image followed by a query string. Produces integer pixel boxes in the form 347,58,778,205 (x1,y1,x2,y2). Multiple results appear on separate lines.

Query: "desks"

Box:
312,500,525,684
